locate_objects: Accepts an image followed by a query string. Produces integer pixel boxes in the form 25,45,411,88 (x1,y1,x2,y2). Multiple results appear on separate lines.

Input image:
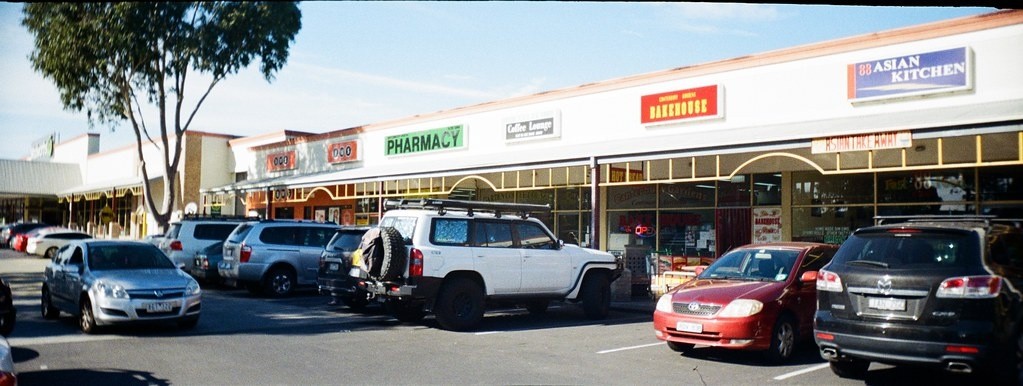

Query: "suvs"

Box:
357,199,623,332
158,217,260,273
813,215,1023,380
217,220,344,297
316,225,374,307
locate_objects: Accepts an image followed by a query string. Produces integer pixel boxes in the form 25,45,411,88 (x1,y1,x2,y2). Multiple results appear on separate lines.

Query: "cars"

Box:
27,230,93,259
192,242,224,285
0,223,48,246
41,240,202,334
652,242,843,364
14,228,74,255
